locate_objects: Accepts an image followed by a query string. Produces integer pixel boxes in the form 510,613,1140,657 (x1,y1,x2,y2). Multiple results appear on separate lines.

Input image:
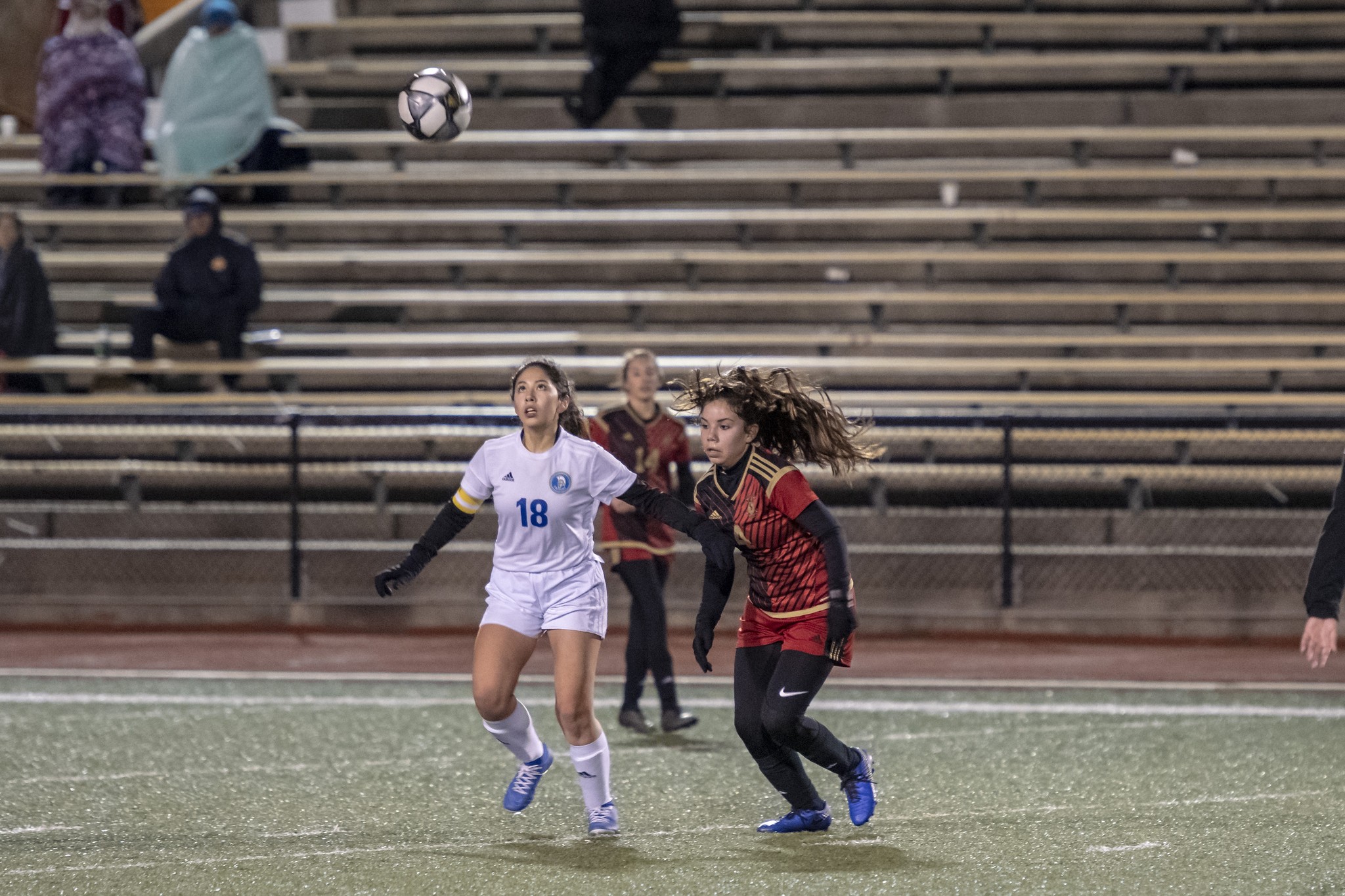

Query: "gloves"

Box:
702,531,734,572
693,626,714,673
825,602,855,641
374,563,418,596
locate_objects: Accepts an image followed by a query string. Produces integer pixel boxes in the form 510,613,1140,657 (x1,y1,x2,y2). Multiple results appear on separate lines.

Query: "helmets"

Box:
183,188,217,219
199,0,237,27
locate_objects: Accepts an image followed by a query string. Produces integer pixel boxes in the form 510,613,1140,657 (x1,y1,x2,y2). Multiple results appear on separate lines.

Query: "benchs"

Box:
0,0,1345,512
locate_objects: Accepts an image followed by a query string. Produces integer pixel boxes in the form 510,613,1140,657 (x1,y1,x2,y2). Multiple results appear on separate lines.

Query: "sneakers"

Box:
838,746,878,826
756,799,831,832
503,744,554,813
586,800,618,834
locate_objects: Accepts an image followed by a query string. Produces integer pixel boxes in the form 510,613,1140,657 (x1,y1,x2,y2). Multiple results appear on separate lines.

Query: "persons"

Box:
669,365,879,833
0,213,59,400
34,0,150,195
152,0,319,208
1300,456,1345,668
580,346,700,731
374,360,734,836
563,0,683,127
125,188,264,390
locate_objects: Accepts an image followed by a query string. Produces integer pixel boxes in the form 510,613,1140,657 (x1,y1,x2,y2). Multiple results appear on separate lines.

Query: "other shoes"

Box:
619,710,655,733
661,711,696,730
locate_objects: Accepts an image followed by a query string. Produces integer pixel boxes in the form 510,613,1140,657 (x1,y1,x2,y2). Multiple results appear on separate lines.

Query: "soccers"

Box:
398,67,473,143
